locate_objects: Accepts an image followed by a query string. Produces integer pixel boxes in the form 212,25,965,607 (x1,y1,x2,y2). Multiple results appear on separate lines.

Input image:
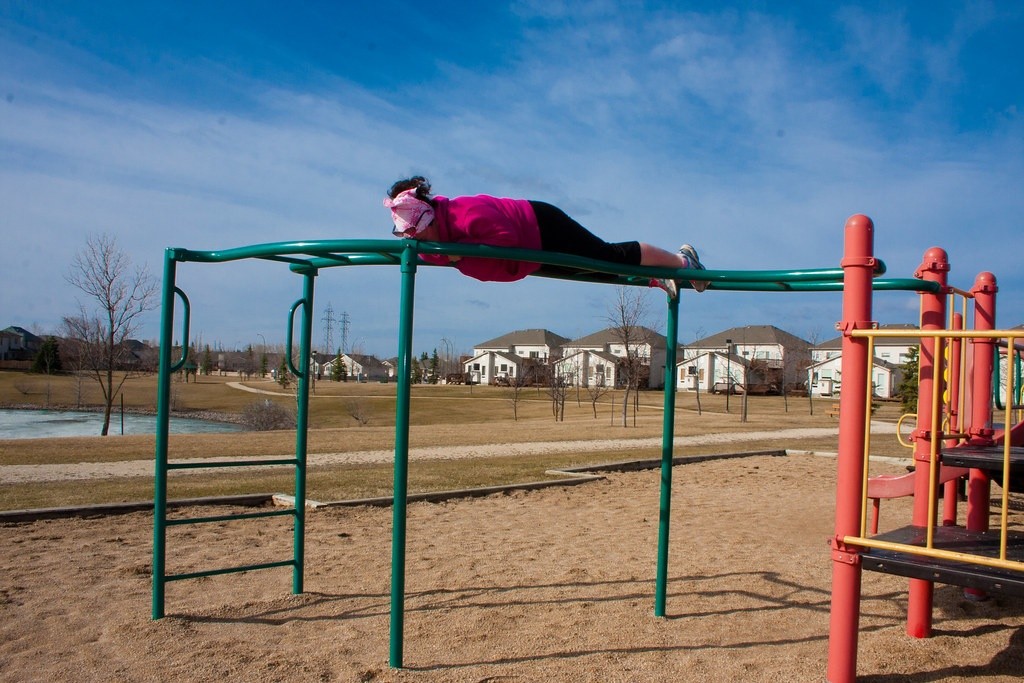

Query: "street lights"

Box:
257,333,266,371
351,336,366,382
726,339,732,412
441,338,453,376
312,351,317,395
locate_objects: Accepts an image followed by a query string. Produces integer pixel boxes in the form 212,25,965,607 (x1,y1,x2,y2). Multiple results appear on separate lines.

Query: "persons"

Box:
383,176,710,298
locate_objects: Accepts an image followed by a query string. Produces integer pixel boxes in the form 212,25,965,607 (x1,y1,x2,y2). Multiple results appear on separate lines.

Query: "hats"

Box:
383,189,434,237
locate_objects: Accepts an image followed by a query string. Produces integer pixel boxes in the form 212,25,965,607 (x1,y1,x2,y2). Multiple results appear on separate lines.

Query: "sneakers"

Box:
679,244,710,292
650,279,678,299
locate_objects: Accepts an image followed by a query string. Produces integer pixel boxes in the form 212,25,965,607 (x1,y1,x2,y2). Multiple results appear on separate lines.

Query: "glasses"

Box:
392,210,430,237
